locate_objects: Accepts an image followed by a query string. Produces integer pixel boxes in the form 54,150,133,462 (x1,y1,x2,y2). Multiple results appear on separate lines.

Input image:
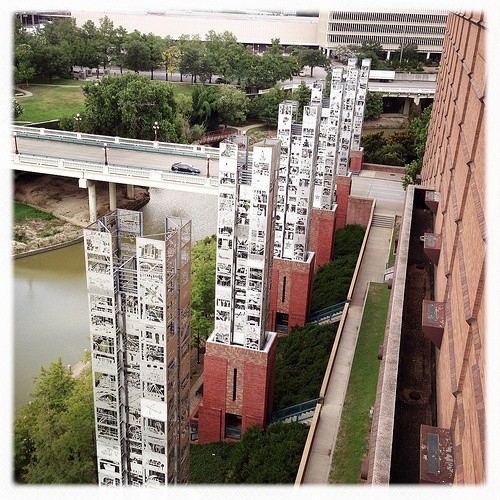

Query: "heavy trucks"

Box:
343,69,395,82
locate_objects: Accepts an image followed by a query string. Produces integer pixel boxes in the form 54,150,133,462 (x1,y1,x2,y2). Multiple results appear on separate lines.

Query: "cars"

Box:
298,72,306,77
215,78,230,84
172,163,200,174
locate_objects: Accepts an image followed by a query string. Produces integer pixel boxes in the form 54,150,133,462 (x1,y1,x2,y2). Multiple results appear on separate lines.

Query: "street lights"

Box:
206,154,212,178
13,132,19,154
102,143,109,165
153,121,159,141
75,113,82,132
